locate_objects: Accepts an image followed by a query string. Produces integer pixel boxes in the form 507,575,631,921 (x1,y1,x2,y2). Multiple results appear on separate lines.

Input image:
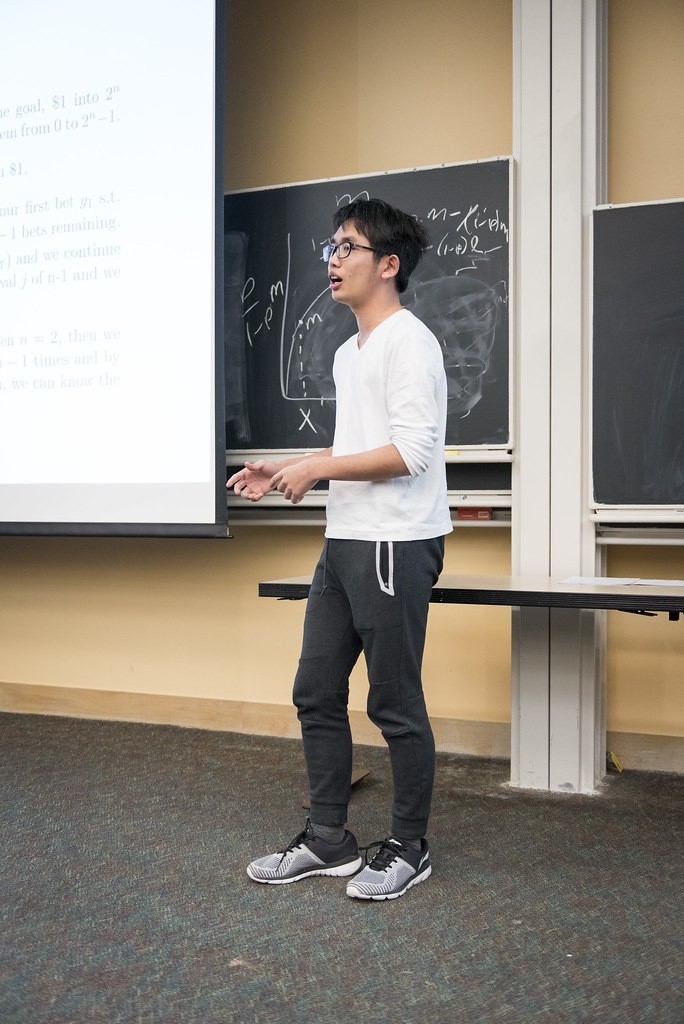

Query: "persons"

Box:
226,200,453,900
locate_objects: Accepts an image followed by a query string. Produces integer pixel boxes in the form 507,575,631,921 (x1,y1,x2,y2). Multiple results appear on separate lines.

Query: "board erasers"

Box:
455,506,493,522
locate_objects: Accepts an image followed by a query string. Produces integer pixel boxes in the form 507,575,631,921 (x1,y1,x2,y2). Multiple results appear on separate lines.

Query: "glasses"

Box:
322,241,385,262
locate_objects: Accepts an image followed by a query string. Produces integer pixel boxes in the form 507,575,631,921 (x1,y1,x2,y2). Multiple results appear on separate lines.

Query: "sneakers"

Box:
246,819,364,885
344,835,434,902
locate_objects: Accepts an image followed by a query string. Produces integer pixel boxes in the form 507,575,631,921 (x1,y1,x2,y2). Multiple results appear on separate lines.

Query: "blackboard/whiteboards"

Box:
225,198,683,546
224,153,517,466
